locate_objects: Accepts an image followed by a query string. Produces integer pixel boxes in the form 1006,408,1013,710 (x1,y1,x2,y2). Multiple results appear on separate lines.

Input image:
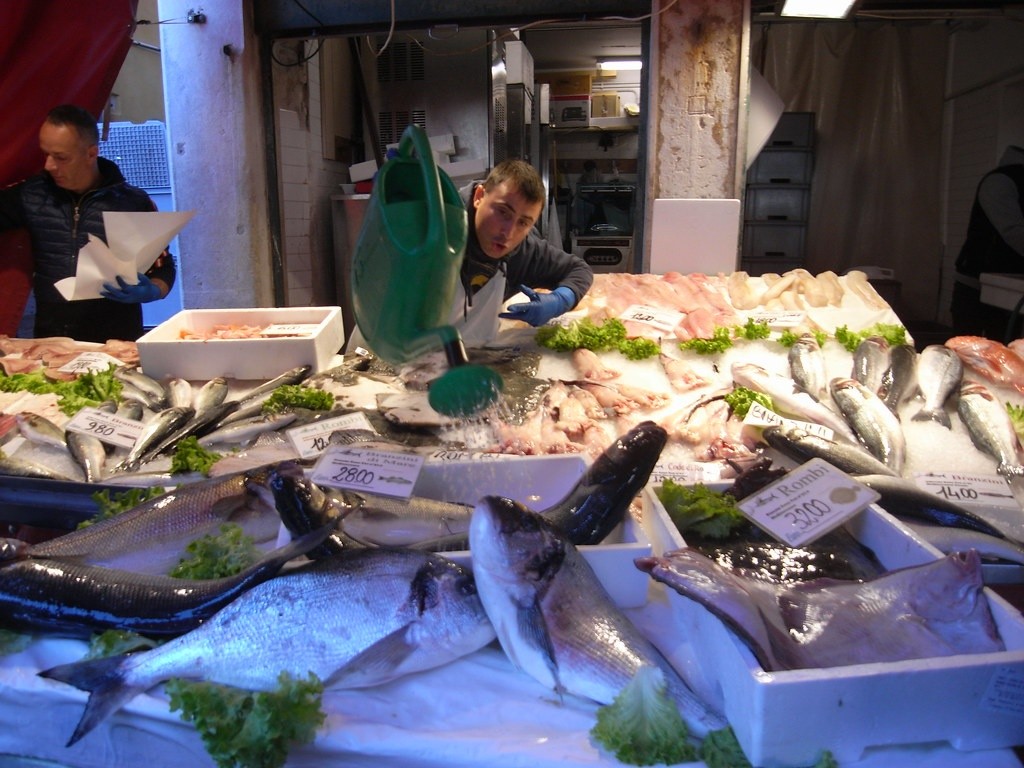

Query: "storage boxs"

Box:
543,74,593,95
643,474,1024,768
348,132,486,193
592,89,621,117
548,96,591,127
505,41,536,95
415,451,652,607
134,305,345,382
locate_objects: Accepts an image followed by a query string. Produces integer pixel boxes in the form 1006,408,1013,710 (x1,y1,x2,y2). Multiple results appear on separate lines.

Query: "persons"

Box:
947,144,1024,346
0,104,176,344
344,158,594,362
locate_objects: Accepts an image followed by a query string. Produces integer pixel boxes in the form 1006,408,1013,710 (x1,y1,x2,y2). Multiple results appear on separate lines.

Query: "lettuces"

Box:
0,361,336,581
88,630,839,768
533,315,1024,538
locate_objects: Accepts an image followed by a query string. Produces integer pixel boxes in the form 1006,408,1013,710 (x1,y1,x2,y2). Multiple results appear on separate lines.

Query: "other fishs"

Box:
730,337,1022,551
572,269,891,343
0,321,374,486
0,419,1023,751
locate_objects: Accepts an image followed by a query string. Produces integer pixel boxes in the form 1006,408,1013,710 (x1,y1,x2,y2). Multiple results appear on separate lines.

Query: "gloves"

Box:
100,272,161,304
498,284,576,327
373,147,397,180
